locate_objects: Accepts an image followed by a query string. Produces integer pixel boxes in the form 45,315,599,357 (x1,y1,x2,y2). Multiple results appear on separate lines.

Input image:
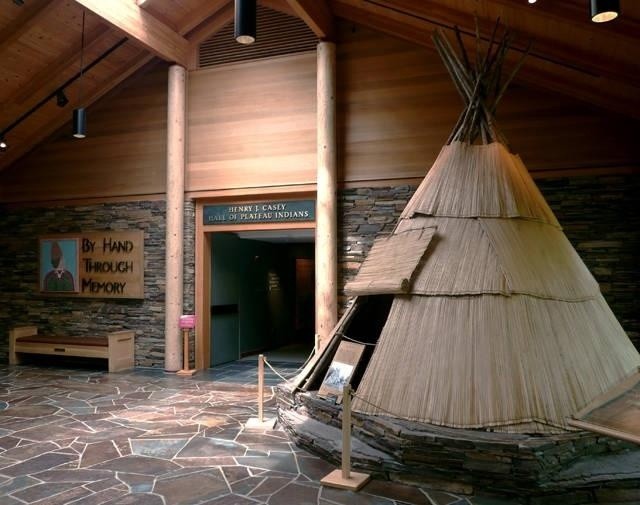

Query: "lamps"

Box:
589,0,618,24
233,0,257,45
72,10,89,139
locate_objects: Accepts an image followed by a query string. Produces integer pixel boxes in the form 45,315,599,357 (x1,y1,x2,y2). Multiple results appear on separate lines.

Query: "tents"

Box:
288,14,640,447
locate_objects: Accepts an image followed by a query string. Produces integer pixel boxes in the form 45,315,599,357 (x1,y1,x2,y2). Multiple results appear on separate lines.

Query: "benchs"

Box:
8,326,135,374
